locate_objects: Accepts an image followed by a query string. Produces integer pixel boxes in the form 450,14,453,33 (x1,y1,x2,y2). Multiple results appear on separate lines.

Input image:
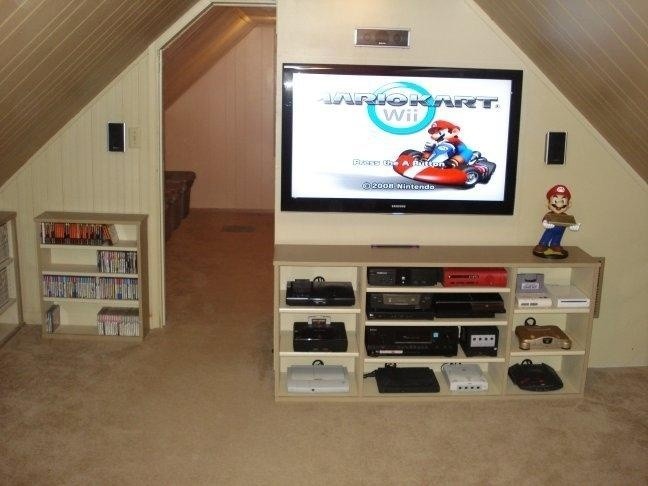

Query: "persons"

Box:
422,119,474,168
533,185,579,259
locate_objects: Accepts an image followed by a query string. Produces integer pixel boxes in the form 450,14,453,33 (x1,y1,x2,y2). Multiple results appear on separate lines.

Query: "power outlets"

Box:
129,127,141,148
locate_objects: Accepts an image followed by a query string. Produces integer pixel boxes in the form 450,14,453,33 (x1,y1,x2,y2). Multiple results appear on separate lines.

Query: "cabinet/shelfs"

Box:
33,211,150,343
0,211,26,346
273,245,601,402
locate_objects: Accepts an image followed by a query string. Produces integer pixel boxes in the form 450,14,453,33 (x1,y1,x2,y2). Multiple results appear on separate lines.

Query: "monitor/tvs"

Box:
281,64,522,215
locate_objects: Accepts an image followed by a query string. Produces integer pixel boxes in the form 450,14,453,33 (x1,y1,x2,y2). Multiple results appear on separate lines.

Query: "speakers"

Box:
544,131,567,162
106,122,123,150
356,29,410,47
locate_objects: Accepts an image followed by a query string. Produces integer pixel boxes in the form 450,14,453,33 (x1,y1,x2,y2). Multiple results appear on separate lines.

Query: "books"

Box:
42,276,138,300
97,250,137,273
96,306,140,337
40,222,118,246
45,304,60,333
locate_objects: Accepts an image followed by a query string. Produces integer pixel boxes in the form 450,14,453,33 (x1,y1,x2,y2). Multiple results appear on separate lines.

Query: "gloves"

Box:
542,220,555,229
570,223,581,231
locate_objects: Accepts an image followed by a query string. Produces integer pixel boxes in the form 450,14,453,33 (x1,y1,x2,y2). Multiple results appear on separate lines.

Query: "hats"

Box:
547,184,571,201
428,120,460,133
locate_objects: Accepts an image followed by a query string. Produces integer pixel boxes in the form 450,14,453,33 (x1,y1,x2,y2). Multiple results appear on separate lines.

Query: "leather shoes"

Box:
533,244,552,258
551,246,563,253
